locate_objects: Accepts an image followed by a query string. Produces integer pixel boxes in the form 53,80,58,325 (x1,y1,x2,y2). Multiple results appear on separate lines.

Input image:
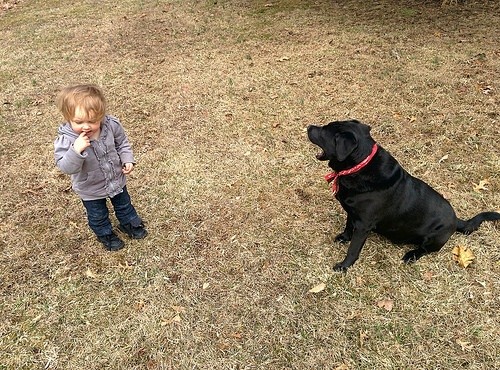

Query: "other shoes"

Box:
95,232,126,252
117,222,148,239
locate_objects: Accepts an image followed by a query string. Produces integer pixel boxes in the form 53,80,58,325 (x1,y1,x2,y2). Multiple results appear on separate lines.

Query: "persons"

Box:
53,83,148,252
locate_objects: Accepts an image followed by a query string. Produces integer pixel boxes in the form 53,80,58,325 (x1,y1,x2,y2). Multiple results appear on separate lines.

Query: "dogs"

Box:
307,119,500,273
